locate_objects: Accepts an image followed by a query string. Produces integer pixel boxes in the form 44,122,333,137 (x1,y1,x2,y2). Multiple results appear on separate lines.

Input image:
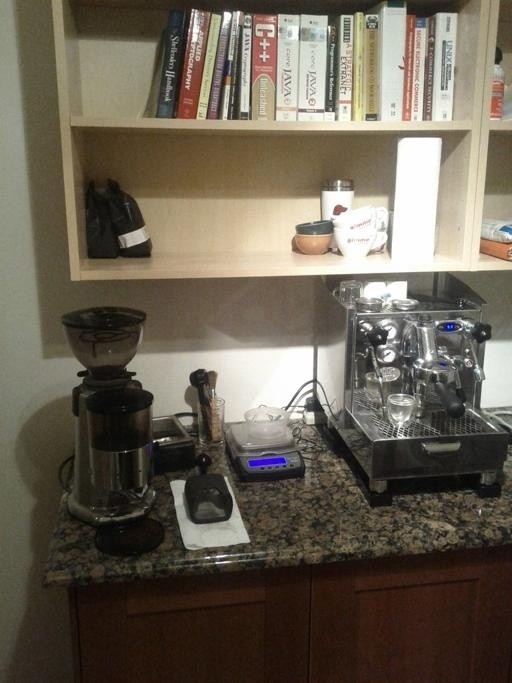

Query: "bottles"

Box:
490,62,505,120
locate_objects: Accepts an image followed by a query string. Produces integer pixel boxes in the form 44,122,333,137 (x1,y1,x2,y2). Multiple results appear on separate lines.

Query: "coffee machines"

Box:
313,282,512,507
58,307,161,527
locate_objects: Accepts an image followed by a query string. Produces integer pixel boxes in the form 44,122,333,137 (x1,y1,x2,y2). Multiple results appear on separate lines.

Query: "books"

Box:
479,217,512,264
157,0,459,122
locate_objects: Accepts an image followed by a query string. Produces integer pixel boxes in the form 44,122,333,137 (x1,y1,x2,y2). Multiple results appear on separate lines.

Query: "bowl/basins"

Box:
243,407,288,438
296,206,389,258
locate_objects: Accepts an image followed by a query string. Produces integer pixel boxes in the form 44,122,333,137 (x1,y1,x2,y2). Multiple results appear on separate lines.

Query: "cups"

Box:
321,179,355,252
339,280,361,308
385,393,416,428
197,399,225,447
364,371,390,403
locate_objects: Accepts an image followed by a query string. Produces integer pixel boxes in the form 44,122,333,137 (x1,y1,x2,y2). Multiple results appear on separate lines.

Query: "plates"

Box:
355,296,420,313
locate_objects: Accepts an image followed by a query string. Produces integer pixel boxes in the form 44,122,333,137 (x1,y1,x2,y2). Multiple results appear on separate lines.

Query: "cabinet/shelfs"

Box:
474,5,511,274
45,0,490,284
39,404,512,682
